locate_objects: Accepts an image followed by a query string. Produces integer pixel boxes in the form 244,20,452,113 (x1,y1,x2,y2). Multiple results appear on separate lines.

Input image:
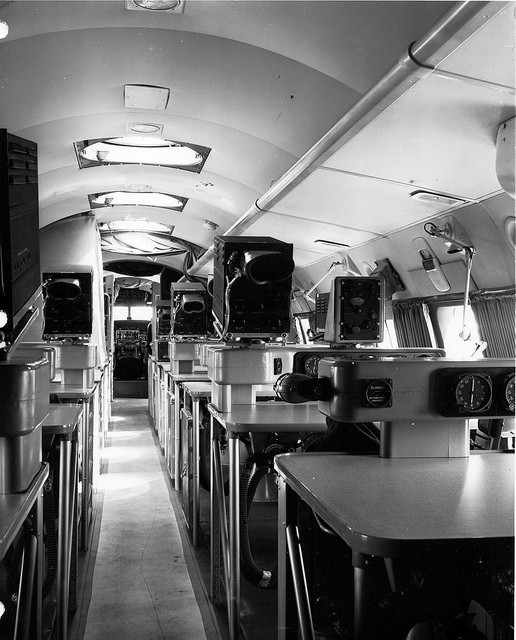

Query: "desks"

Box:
179,380,275,547
1,459,54,638
49,379,96,553
206,396,328,639
271,449,514,640
157,363,172,455
40,402,85,638
165,370,212,492
